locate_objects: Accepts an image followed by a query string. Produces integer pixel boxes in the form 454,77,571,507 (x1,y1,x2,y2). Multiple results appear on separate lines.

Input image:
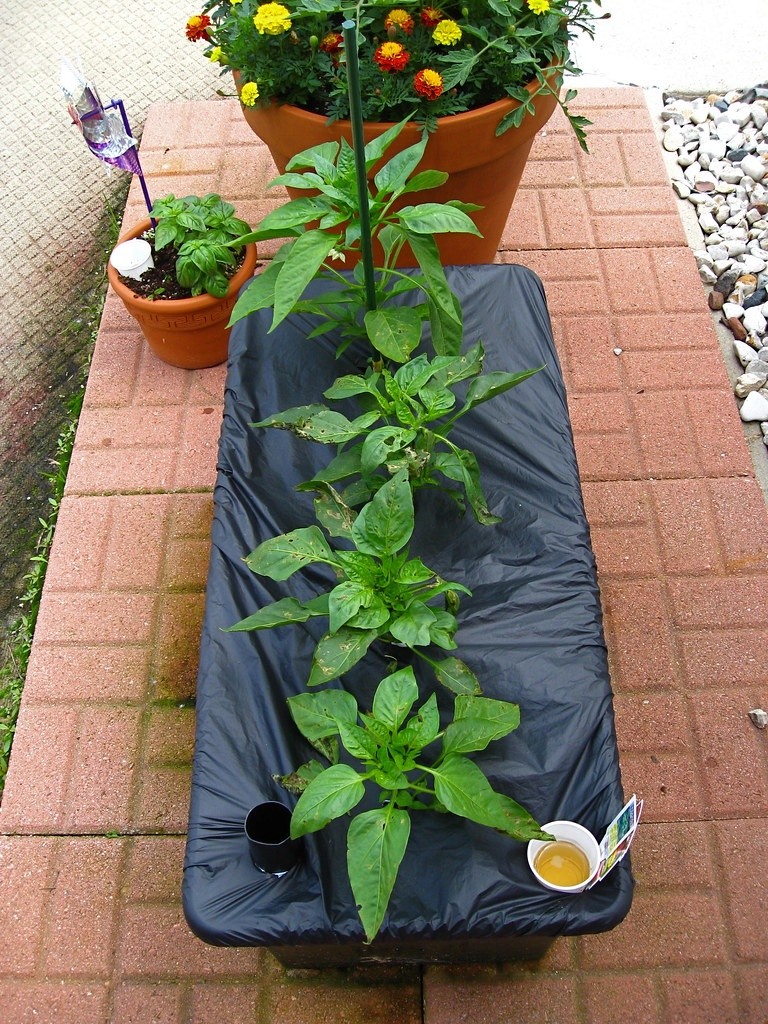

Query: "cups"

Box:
244,801,298,873
110,239,154,282
527,820,600,891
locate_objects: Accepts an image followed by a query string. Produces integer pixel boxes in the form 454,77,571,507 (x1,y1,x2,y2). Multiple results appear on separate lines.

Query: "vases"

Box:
232,21,566,272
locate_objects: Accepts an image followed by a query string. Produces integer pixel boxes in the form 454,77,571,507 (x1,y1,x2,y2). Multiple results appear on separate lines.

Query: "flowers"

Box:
187,0,612,156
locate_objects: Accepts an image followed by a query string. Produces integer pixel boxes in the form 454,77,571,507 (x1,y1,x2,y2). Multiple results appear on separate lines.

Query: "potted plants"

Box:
108,195,257,370
180,105,634,973
